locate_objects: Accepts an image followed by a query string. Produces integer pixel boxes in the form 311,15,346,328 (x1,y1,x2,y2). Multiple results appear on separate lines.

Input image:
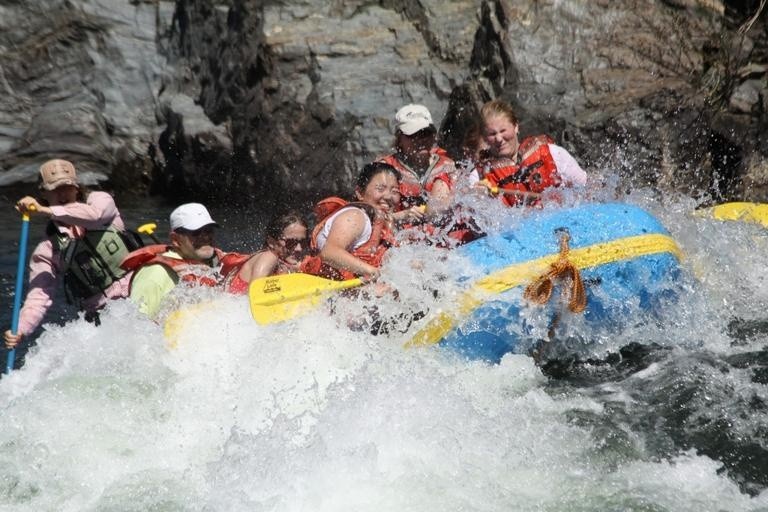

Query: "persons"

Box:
4,158,135,349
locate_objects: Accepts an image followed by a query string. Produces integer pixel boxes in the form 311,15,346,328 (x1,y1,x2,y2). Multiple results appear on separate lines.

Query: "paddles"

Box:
248,274,365,325
484,179,768,233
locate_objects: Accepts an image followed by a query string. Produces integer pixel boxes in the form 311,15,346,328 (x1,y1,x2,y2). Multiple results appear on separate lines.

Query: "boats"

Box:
164,202,683,366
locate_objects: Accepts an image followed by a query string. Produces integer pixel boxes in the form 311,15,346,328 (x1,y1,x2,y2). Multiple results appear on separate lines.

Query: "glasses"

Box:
175,227,215,238
274,236,312,250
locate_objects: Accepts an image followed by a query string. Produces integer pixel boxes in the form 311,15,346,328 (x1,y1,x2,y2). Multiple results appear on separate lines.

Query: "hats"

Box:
170,203,220,235
394,103,433,137
36,157,79,193
